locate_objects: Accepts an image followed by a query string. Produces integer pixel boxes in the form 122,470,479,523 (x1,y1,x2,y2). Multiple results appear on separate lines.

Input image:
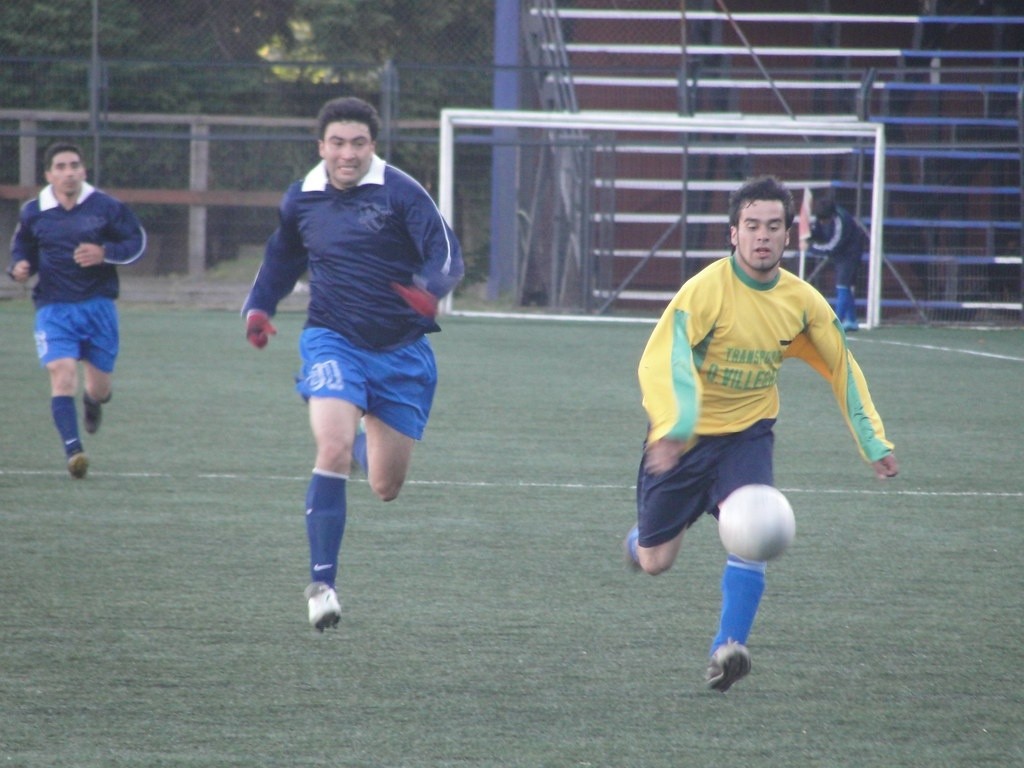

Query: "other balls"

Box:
716,482,797,561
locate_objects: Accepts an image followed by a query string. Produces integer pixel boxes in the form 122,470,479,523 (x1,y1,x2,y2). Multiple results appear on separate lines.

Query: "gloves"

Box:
391,281,440,320
244,308,278,349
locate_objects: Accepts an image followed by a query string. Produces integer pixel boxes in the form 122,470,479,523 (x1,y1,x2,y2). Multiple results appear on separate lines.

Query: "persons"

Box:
243,95,465,635
623,173,898,693
804,198,863,332
7,141,147,483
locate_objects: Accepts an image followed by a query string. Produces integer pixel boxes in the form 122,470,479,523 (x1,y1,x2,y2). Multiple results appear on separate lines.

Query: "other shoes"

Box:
68,453,87,481
705,636,752,693
82,403,101,434
303,581,342,633
623,523,641,572
